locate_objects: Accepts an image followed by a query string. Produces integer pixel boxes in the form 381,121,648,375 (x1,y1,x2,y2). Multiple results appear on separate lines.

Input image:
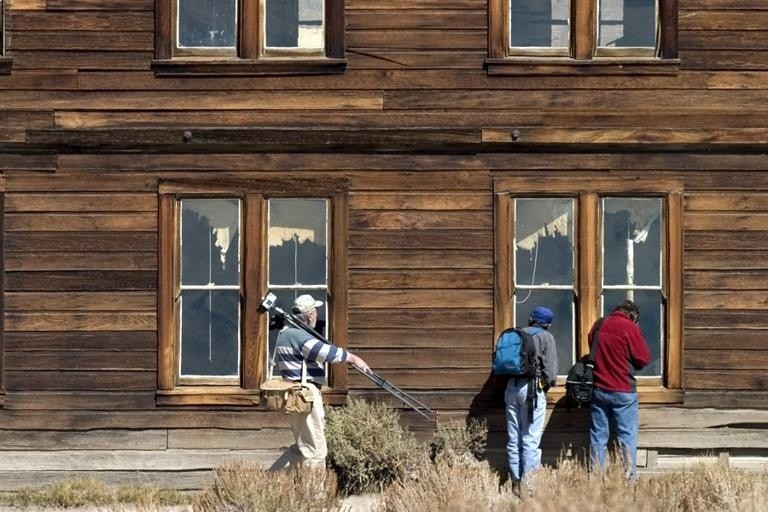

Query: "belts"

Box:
294,378,321,389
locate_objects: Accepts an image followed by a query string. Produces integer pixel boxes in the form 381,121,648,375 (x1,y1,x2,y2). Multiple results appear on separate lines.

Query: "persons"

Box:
502,306,558,484
276,293,373,501
588,299,651,483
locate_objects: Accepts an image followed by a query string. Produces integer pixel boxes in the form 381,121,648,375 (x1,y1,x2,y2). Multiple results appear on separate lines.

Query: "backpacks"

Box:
493,325,543,375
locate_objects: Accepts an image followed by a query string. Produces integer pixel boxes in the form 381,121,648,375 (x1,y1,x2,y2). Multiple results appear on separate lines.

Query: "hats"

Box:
292,294,324,315
531,305,553,323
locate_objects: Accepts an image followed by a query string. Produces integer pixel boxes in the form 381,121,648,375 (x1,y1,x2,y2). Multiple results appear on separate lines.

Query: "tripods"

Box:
275,306,433,420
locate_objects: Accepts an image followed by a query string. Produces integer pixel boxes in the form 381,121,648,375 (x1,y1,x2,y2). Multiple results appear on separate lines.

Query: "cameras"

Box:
255,291,277,315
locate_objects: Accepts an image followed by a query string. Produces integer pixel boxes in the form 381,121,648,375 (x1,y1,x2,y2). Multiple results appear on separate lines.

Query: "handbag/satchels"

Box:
259,379,314,415
567,354,594,404
